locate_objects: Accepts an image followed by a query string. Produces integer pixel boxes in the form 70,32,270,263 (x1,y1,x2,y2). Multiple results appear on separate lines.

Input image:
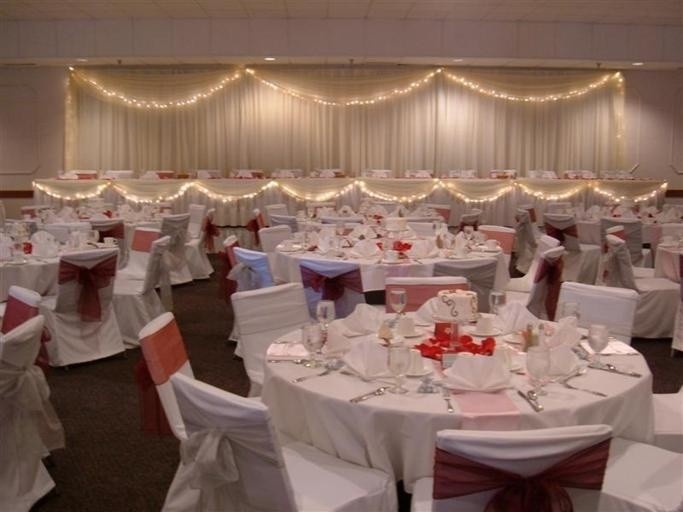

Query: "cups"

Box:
473,313,496,333
486,240,500,250
103,236,118,247
385,250,399,261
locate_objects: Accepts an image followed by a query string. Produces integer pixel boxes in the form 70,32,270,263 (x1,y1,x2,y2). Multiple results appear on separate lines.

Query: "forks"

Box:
561,383,606,397
292,369,330,385
442,389,453,413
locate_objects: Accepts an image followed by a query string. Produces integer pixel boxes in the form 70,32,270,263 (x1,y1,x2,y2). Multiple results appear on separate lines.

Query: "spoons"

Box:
349,387,385,403
526,389,541,411
606,363,640,378
267,359,301,364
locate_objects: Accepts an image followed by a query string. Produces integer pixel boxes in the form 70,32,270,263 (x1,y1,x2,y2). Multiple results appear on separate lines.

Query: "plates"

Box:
469,330,500,336
402,368,432,378
404,330,426,338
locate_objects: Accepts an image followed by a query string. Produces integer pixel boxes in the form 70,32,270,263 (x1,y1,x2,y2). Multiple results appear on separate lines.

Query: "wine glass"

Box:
486,289,505,317
300,324,326,368
389,288,407,316
561,301,580,326
524,345,547,396
316,300,335,334
586,322,608,370
386,345,408,394
